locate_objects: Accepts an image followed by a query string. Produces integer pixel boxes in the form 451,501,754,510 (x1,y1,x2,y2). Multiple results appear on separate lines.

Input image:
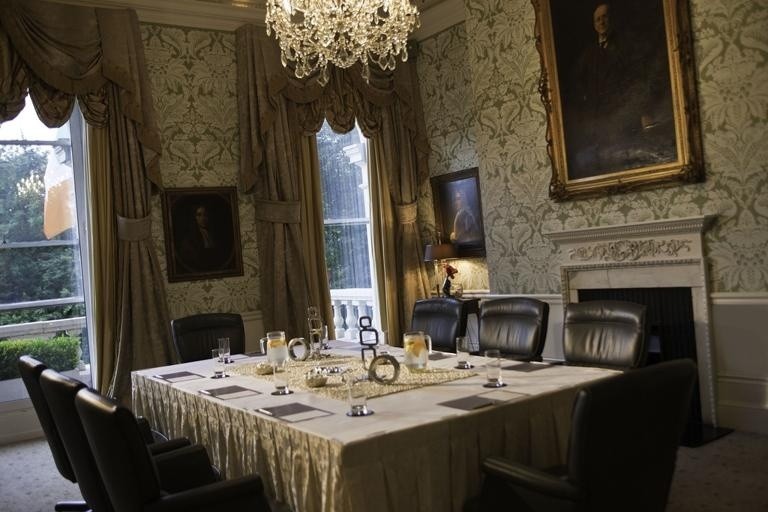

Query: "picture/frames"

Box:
160,185,245,283
430,166,487,261
531,0,707,203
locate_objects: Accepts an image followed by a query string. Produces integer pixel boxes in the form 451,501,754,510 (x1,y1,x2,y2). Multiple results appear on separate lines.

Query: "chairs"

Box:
40,368,193,512
409,299,469,354
482,358,699,512
561,301,649,369
18,354,113,511
170,312,246,363
469,297,549,361
75,387,272,512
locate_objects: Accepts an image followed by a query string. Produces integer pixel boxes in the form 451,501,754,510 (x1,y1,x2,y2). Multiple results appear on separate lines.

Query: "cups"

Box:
219,338,231,364
485,350,502,387
347,377,370,414
212,349,225,379
457,337,471,369
272,360,290,393
322,325,329,350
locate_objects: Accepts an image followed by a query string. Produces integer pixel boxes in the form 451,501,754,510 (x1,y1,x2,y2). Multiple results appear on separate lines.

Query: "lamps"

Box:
424,243,464,302
266,1,421,88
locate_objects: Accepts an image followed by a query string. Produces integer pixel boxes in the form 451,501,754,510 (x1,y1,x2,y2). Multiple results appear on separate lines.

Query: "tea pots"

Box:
259,331,288,367
403,331,432,372
